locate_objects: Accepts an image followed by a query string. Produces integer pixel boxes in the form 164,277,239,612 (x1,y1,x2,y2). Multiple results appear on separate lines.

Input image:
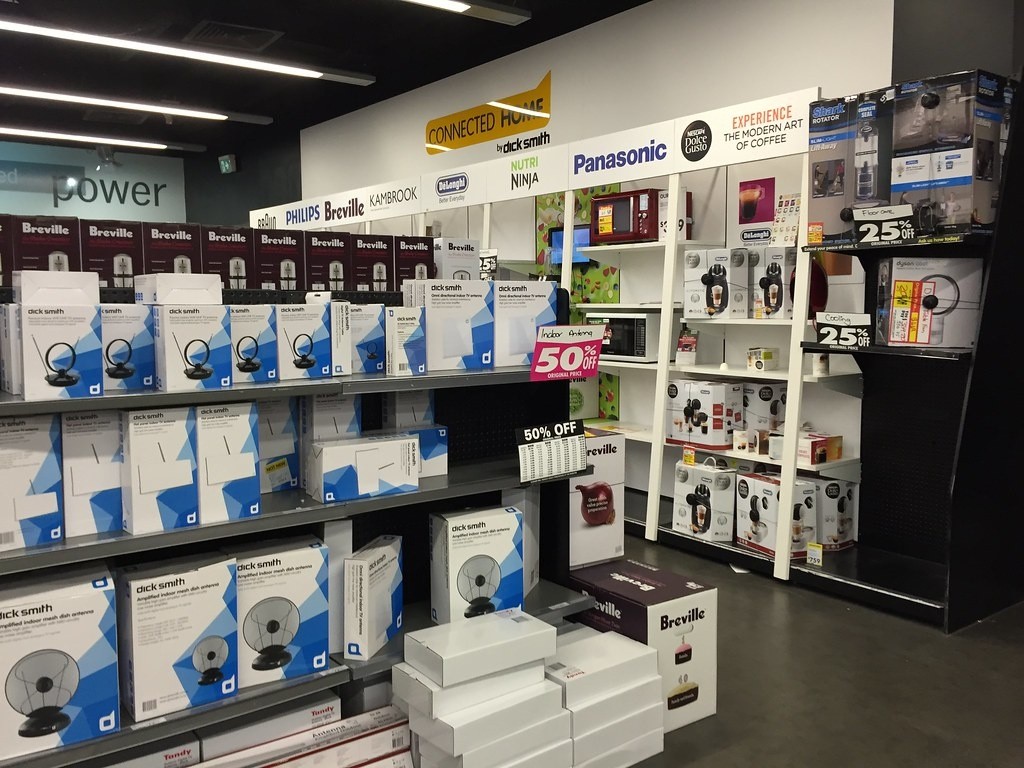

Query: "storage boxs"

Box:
0,69,1024,768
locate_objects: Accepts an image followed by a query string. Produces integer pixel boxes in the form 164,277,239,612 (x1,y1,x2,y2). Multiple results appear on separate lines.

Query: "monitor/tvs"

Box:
548,224,597,266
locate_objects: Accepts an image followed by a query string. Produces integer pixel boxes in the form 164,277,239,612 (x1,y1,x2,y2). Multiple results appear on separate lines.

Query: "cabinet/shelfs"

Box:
354,148,867,578
0,359,598,768
799,81,1024,628
